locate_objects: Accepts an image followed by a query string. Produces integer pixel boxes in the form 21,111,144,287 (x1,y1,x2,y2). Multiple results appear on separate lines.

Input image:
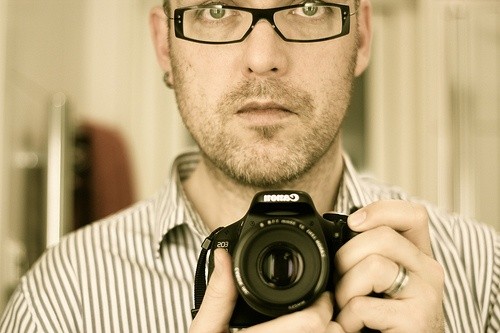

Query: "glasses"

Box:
163,2,365,45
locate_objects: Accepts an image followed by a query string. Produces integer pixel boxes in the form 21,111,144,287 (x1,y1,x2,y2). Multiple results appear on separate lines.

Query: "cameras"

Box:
207,190,384,333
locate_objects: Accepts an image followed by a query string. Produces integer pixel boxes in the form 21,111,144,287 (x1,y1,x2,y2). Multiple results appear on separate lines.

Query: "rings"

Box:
382,262,412,298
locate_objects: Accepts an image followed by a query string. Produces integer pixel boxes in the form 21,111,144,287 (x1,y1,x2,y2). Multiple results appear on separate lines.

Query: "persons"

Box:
1,0,500,333
